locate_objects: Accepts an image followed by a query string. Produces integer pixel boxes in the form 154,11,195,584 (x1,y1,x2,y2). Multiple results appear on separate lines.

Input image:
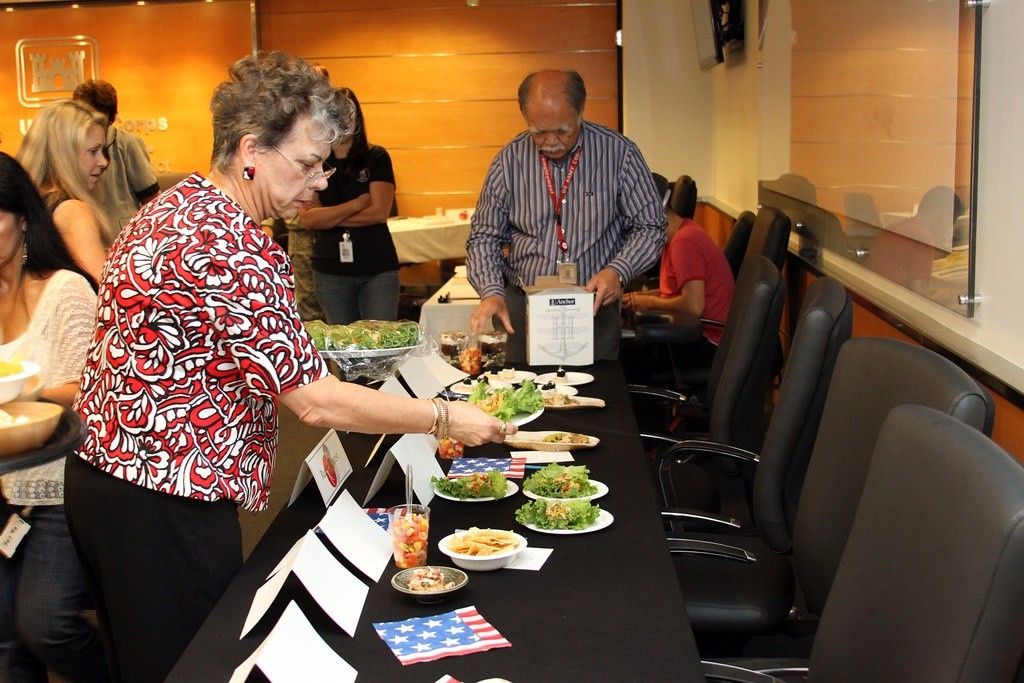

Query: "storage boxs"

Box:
518,275,596,368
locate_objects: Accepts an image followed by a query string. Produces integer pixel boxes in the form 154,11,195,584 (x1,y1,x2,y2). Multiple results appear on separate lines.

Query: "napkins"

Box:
447,456,527,479
510,449,575,464
434,674,463,683
315,507,408,532
502,547,555,572
372,605,512,667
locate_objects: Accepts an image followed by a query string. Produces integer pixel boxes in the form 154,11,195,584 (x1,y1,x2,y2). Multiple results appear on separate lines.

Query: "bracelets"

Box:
427,398,449,441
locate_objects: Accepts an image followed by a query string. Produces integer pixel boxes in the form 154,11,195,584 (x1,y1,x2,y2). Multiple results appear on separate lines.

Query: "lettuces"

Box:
430,468,507,501
466,379,544,423
522,460,598,499
514,498,602,531
303,319,420,350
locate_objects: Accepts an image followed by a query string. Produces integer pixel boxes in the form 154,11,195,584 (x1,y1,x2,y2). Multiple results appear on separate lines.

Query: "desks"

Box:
386,208,476,265
418,272,494,351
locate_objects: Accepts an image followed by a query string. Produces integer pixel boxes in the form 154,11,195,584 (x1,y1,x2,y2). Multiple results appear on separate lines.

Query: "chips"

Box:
449,527,519,556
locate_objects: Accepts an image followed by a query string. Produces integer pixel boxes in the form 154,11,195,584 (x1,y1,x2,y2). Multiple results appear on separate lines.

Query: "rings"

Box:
499,422,507,435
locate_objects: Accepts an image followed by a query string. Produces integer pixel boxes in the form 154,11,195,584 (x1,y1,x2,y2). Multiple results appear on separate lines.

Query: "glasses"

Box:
269,145,337,186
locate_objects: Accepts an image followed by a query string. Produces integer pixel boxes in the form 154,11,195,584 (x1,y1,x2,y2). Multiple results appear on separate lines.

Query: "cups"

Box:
436,208,443,216
385,504,431,570
438,331,508,375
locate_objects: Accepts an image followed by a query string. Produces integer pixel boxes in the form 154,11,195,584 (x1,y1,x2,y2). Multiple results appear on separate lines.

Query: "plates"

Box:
545,397,605,411
524,509,614,534
318,335,427,359
504,430,600,452
433,478,519,502
508,406,546,426
522,479,609,502
477,370,537,385
449,380,513,396
534,372,594,385
516,385,579,396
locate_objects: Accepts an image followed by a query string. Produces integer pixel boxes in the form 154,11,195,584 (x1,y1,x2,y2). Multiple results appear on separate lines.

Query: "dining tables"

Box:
162,358,707,683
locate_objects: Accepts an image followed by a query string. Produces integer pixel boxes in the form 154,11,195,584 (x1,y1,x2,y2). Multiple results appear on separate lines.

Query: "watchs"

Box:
619,276,624,288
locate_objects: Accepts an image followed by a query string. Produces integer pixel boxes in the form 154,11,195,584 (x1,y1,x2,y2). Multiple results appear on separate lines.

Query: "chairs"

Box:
666,204,794,390
661,333,993,634
625,253,788,480
719,210,755,284
656,276,856,552
699,400,1024,683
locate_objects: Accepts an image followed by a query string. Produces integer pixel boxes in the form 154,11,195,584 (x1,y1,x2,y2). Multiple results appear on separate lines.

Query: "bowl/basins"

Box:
0,359,66,458
438,529,529,572
391,565,470,604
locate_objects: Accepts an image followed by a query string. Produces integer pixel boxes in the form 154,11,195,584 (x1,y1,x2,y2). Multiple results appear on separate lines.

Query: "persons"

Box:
261,86,402,325
63,51,518,683
14,98,115,285
0,151,101,683
73,79,162,239
865,185,964,295
464,66,669,363
621,173,736,458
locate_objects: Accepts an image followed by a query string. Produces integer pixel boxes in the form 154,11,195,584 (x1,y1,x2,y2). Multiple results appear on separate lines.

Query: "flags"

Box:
371,606,512,666
447,457,526,479
314,508,407,537
